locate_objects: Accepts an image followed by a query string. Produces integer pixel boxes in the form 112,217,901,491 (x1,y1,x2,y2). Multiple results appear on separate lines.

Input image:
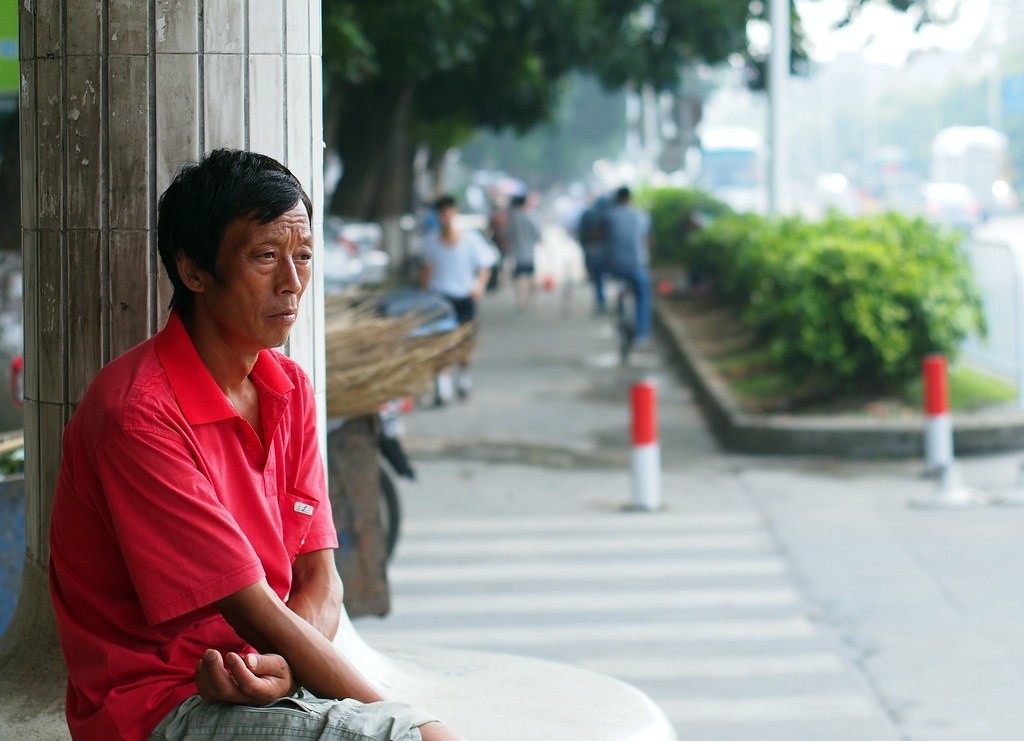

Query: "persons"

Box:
416,197,494,406
585,186,652,351
484,201,510,291
48,148,466,741
505,195,544,312
569,195,612,312
